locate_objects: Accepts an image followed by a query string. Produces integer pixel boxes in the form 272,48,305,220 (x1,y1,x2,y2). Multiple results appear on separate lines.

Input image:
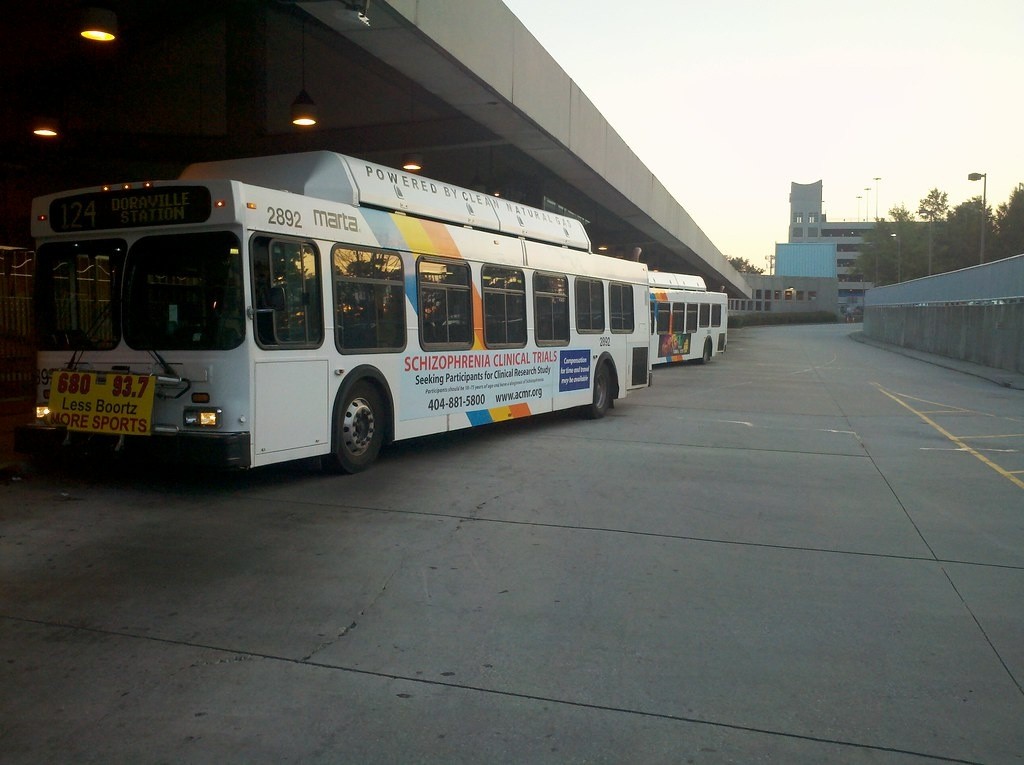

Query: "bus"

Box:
648,271,729,365
28,149,651,476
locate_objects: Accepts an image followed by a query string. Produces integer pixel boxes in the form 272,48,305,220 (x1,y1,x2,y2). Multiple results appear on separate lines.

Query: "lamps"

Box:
290,19,319,127
402,81,423,171
78,0,119,42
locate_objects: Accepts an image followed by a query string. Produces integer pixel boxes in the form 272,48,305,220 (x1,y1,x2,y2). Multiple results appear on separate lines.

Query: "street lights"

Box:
873,178,880,221
968,172,986,264
864,187,871,222
855,196,862,223
920,210,933,276
891,233,901,283
